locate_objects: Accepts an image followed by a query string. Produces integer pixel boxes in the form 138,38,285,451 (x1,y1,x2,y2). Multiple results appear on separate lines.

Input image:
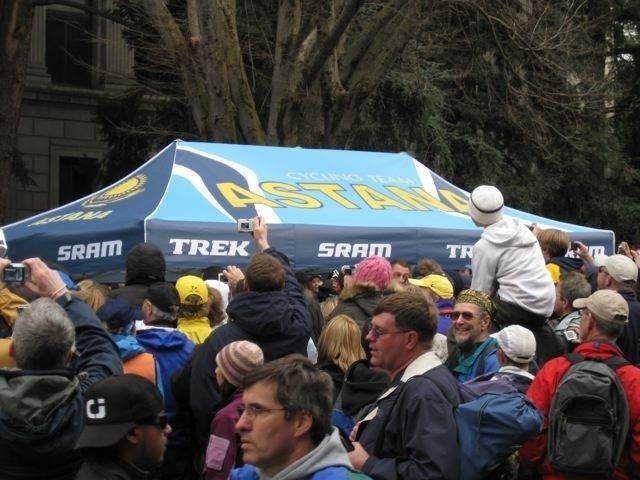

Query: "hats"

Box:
407,274,453,300
489,324,536,363
135,282,181,313
352,256,392,291
215,340,264,387
0,228,81,291
572,290,629,325
72,373,165,450
596,254,638,287
175,276,209,305
468,185,504,225
97,302,145,330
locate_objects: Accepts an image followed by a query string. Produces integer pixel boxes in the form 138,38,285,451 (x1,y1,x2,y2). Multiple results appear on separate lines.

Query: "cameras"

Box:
1,262,32,282
238,218,255,233
569,241,578,251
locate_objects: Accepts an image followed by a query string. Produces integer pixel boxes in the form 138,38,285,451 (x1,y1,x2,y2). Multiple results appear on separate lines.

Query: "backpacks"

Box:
549,352,632,477
372,374,545,480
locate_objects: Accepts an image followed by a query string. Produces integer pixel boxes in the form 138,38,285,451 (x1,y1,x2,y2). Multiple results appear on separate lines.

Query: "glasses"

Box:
367,323,414,339
237,404,288,422
598,264,610,276
578,309,591,318
149,413,169,430
450,311,479,321
